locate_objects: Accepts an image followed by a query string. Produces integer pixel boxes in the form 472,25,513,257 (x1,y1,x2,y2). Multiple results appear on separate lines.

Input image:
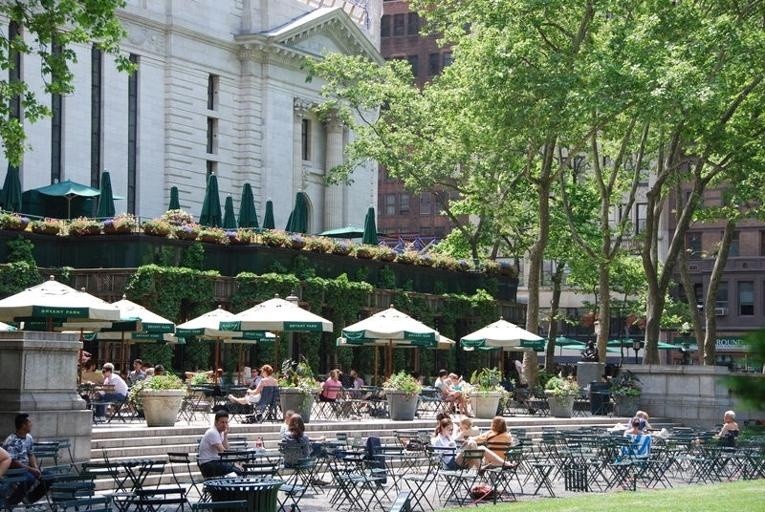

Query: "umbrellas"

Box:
176,305,280,373
218,293,333,373
336,335,456,375
545,336,682,362
341,303,440,376
1,275,188,354
460,316,545,370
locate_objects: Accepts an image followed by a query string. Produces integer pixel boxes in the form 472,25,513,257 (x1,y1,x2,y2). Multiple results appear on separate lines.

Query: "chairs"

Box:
0,381,765,512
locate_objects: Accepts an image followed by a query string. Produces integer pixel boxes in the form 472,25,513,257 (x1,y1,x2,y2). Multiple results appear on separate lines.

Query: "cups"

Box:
234,376,239,386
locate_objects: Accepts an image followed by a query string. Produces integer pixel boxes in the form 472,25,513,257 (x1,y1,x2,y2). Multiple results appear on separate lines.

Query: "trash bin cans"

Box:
201,477,286,512
589,379,612,415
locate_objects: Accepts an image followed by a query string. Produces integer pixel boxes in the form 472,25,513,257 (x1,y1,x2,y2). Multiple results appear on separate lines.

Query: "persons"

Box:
354,370,422,410
183,367,278,417
718,410,739,452
199,409,246,476
315,368,365,400
613,410,652,464
81,357,165,425
1,412,53,512
430,368,537,474
280,408,331,487
0,447,12,476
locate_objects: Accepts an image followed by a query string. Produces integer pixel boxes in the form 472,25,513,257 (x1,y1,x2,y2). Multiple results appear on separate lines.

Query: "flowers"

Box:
0,209,469,271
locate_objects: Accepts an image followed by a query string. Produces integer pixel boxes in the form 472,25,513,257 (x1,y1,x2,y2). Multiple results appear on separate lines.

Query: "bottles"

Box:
256,437,263,449
480,430,484,435
